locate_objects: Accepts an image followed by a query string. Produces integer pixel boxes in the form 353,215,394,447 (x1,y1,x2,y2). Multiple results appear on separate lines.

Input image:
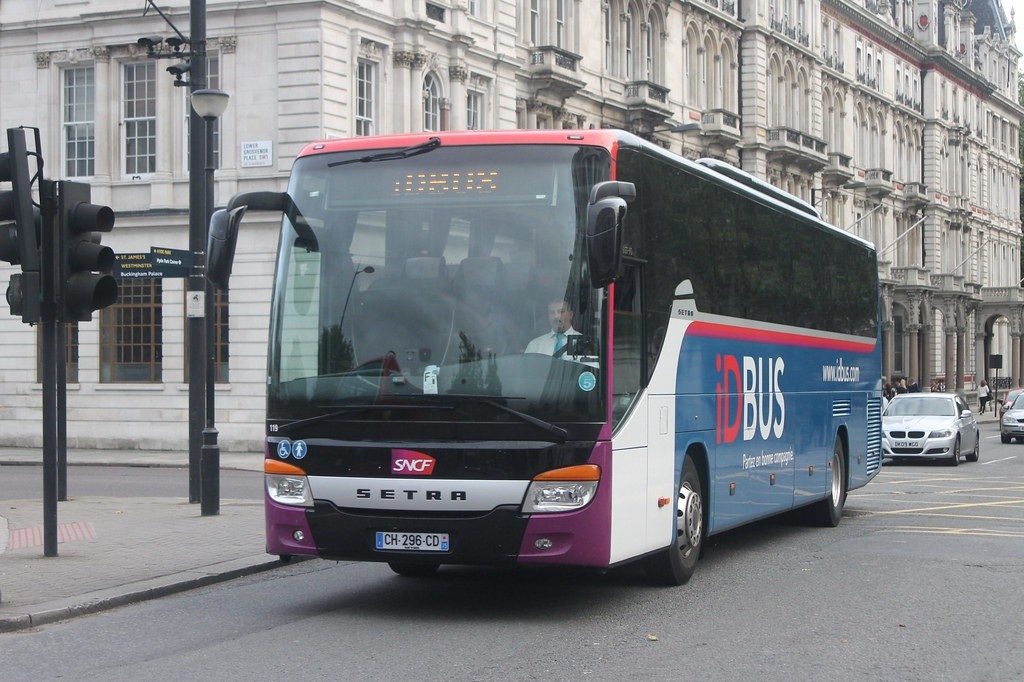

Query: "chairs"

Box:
404,253,551,336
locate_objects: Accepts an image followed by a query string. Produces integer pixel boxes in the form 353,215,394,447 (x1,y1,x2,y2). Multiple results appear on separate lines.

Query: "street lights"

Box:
188,85,230,517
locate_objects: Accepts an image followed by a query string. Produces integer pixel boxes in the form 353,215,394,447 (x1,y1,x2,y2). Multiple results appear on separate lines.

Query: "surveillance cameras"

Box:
137,35,187,46
167,63,190,75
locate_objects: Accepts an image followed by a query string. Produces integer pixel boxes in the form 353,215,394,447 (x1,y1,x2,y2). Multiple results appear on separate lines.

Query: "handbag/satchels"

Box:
986,393,993,401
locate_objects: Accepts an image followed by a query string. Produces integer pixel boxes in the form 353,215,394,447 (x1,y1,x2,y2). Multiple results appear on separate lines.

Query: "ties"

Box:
553,334,564,359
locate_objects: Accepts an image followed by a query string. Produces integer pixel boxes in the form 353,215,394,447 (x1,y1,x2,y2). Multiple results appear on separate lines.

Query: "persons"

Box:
883,379,918,401
524,301,600,369
978,380,989,415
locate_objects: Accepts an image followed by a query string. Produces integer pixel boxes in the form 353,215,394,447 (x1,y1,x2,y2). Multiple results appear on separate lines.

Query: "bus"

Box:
204,128,888,585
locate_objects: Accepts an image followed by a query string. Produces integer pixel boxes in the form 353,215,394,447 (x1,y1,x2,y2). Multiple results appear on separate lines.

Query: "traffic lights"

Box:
32,206,42,248
0,126,40,271
5,271,40,324
58,179,120,322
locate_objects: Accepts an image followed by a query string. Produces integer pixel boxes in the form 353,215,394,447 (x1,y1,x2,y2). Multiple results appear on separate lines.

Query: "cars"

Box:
997,388,1024,430
1000,392,1024,444
882,392,980,466
